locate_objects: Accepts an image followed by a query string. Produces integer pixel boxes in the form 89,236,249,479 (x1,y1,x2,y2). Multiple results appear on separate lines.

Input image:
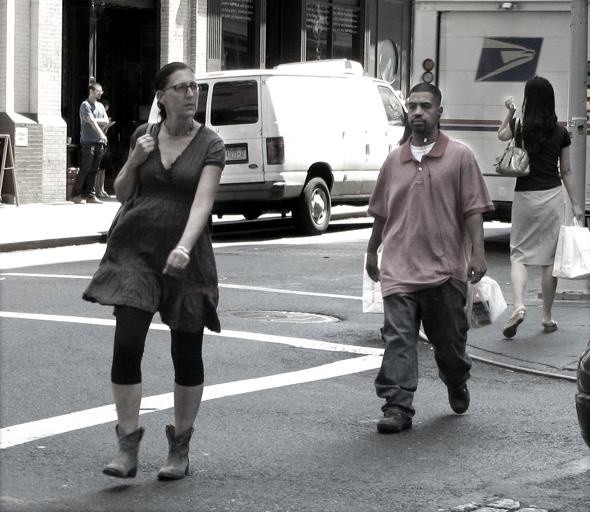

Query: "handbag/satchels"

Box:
553,215,590,281
105,122,156,242
362,242,384,315
494,118,531,178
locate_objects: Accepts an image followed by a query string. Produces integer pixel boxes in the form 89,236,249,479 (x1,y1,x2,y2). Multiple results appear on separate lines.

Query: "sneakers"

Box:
446,383,471,415
375,408,413,434
69,190,110,204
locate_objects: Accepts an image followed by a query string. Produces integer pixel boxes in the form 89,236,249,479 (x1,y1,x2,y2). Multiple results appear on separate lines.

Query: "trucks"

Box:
409,1,590,234
147,58,409,236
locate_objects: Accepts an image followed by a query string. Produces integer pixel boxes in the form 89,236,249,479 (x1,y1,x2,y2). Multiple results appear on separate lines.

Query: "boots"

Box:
103,423,144,479
155,423,195,482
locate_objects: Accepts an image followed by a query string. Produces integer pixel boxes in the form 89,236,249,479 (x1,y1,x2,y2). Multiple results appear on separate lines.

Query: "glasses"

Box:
95,91,103,94
162,81,198,92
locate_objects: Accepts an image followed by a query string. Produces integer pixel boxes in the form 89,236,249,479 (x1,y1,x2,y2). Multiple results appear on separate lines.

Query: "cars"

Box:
574,337,590,448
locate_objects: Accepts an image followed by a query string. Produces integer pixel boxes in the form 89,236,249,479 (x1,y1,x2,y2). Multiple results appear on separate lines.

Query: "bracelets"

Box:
173,243,192,258
366,251,378,257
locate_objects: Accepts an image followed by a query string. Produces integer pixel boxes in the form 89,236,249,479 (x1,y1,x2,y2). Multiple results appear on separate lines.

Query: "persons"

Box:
364,82,497,434
496,73,583,340
94,100,122,198
70,80,111,206
378,108,443,343
82,59,229,480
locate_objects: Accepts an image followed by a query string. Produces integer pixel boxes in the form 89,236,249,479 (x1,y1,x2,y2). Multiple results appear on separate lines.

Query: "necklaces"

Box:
161,116,195,137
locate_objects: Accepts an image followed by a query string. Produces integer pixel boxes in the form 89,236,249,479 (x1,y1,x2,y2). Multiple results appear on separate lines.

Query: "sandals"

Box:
503,305,527,339
541,318,558,333
